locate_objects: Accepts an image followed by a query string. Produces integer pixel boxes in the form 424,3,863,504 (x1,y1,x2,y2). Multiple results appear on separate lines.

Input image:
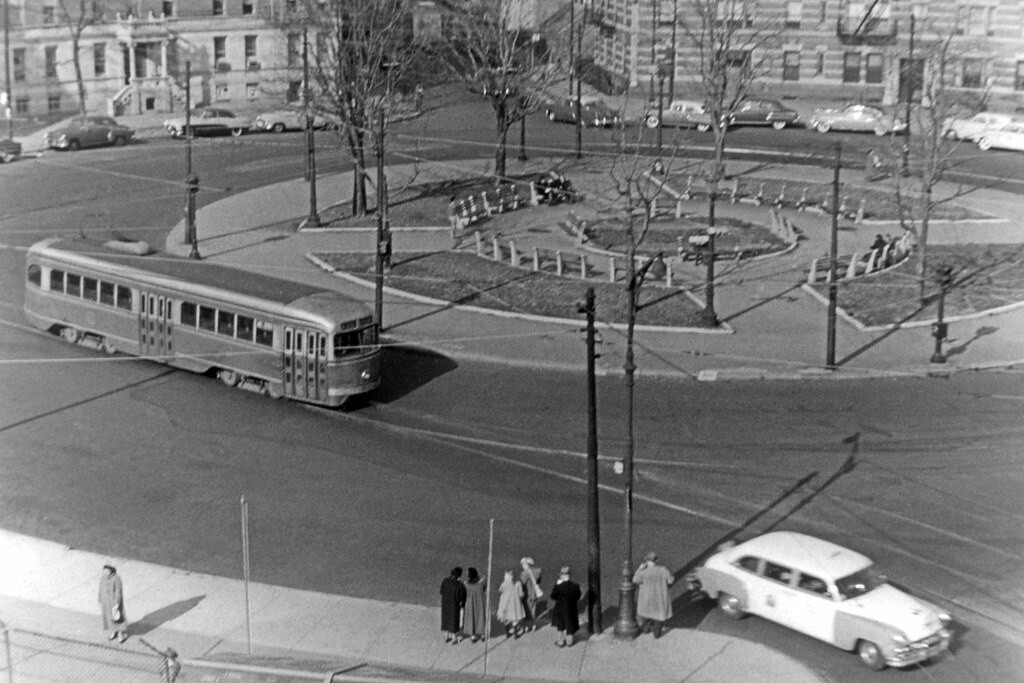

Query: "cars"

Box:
47,114,137,152
810,103,908,138
698,530,957,671
720,96,800,131
163,105,252,139
254,99,342,134
546,92,623,127
940,109,1024,151
643,98,712,133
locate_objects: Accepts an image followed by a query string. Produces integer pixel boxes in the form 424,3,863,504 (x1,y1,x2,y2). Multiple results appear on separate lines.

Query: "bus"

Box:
22,230,382,408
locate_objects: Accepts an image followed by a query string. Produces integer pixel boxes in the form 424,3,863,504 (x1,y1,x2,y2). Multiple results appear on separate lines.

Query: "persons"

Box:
846,574,872,591
439,567,467,645
462,568,488,642
520,556,545,632
413,80,424,110
652,255,667,280
98,561,128,644
870,233,893,257
497,569,526,640
549,567,582,647
632,551,675,638
539,175,575,206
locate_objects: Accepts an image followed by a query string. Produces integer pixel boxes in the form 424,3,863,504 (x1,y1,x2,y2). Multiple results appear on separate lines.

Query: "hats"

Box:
452,567,463,576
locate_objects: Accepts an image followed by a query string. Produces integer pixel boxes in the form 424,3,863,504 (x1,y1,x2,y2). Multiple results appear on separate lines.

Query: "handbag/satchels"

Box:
535,584,543,599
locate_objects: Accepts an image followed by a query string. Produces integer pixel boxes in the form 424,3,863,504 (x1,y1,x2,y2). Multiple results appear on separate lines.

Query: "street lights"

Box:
613,223,669,640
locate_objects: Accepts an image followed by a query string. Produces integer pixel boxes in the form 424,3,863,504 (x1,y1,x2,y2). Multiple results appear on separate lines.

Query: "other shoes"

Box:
556,640,563,649
